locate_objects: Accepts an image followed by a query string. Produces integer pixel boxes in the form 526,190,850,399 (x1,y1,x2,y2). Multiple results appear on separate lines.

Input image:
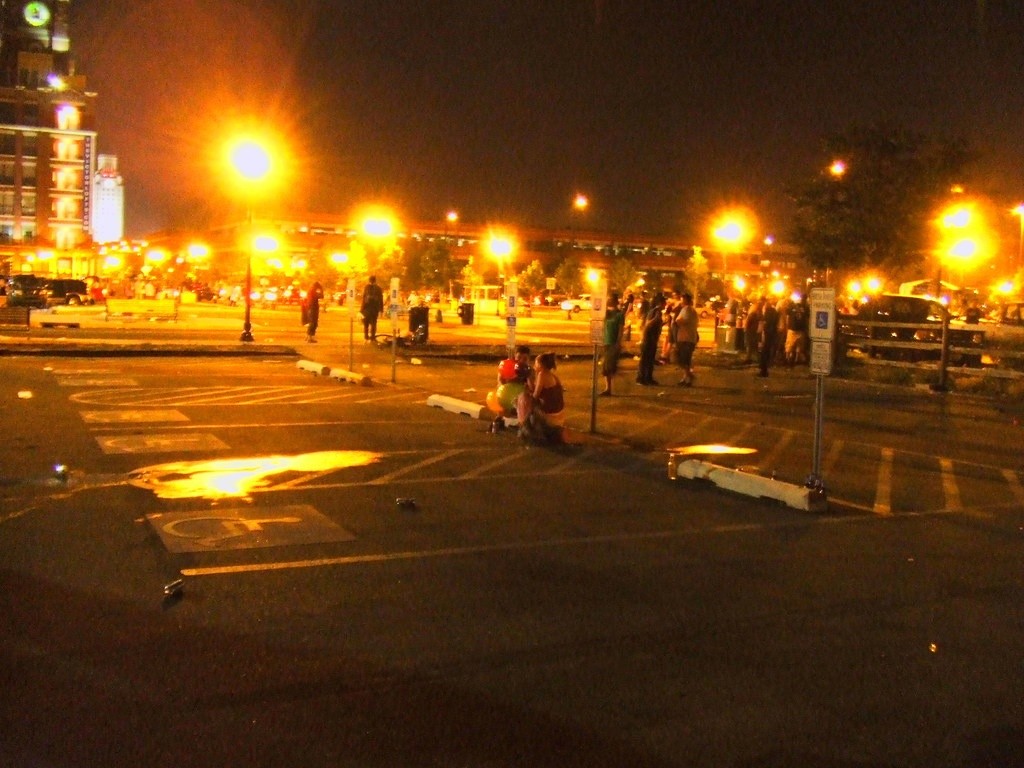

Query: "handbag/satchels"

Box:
539,395,563,412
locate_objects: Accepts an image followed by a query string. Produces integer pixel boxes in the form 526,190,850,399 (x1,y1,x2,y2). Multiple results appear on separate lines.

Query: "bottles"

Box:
668,453,677,480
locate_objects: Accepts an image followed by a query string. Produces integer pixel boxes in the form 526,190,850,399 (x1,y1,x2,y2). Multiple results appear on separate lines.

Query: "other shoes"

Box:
635,379,659,386
756,371,769,377
598,389,611,397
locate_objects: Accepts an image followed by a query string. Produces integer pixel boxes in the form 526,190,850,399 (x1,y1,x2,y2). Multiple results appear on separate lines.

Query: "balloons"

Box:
485,359,535,412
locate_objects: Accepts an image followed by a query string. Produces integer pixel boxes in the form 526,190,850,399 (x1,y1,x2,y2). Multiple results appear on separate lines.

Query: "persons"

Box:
303,281,323,344
598,296,624,396
359,276,383,341
834,289,1003,323
515,351,564,440
625,289,809,386
487,345,535,434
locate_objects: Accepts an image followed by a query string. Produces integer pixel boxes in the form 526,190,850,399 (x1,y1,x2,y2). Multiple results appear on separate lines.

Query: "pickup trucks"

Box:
837,296,983,362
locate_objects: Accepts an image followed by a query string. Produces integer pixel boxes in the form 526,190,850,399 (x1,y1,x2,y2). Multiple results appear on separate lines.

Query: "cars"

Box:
333,290,346,306
562,294,593,314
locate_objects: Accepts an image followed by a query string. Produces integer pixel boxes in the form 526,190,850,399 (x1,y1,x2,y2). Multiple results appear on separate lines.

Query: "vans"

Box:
6,275,38,304
450,285,530,317
32,278,89,306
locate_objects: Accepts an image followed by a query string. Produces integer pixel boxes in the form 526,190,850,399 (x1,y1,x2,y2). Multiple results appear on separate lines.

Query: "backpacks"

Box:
521,410,562,446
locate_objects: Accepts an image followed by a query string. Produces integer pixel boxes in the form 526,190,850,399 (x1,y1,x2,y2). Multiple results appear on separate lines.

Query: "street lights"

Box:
443,211,458,301
226,136,270,339
715,216,741,278
